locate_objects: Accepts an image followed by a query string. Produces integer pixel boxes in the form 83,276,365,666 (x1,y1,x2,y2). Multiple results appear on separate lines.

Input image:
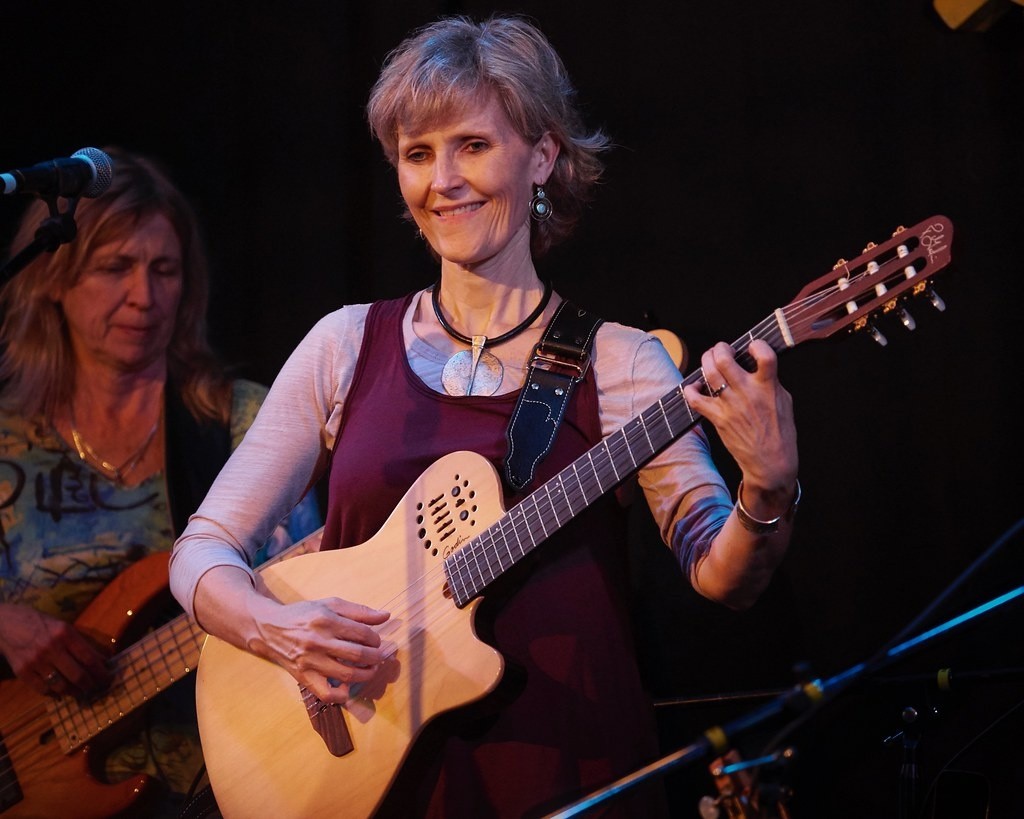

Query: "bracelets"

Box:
737,479,803,535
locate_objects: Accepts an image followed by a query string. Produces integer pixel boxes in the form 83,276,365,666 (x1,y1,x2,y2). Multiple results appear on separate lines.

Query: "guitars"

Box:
196,214,955,819
0,328,688,819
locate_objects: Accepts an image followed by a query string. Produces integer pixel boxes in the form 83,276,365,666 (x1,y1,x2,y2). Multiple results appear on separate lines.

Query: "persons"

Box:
0,150,324,819
170,16,800,819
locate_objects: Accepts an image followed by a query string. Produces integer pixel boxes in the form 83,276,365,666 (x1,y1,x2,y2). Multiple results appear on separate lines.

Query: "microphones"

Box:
0,146,115,198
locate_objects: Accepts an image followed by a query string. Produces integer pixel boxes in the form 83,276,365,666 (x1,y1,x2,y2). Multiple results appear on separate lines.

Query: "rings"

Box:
47,671,61,683
713,384,727,396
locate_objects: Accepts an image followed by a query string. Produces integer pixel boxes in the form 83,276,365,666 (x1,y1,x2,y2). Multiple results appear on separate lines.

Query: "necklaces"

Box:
66,393,166,486
431,275,553,393
